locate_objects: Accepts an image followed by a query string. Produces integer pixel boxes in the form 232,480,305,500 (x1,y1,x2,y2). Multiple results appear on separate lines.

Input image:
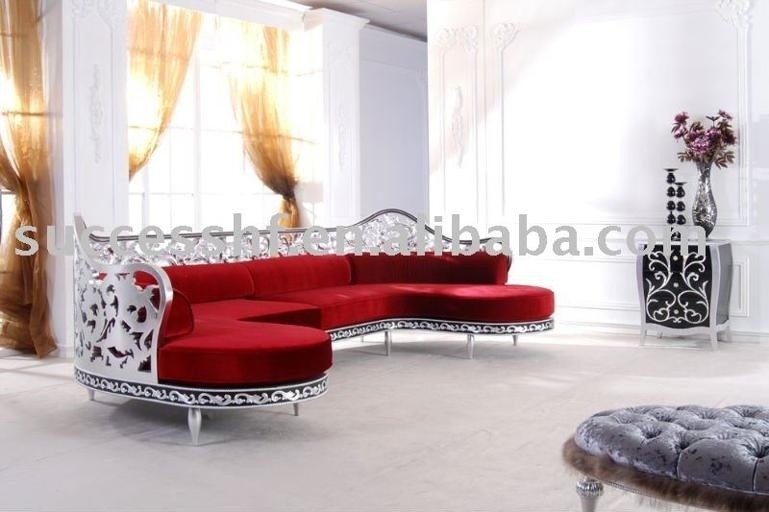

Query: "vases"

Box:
692,163,716,238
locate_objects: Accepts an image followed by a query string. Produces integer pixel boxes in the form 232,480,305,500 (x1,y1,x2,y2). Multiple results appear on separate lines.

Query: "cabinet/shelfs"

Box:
635,239,733,349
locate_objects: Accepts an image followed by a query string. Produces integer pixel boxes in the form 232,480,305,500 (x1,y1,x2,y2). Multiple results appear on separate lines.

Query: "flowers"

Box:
673,108,736,170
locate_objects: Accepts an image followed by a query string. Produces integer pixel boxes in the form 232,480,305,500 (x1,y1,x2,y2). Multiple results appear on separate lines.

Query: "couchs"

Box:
71,208,555,447
